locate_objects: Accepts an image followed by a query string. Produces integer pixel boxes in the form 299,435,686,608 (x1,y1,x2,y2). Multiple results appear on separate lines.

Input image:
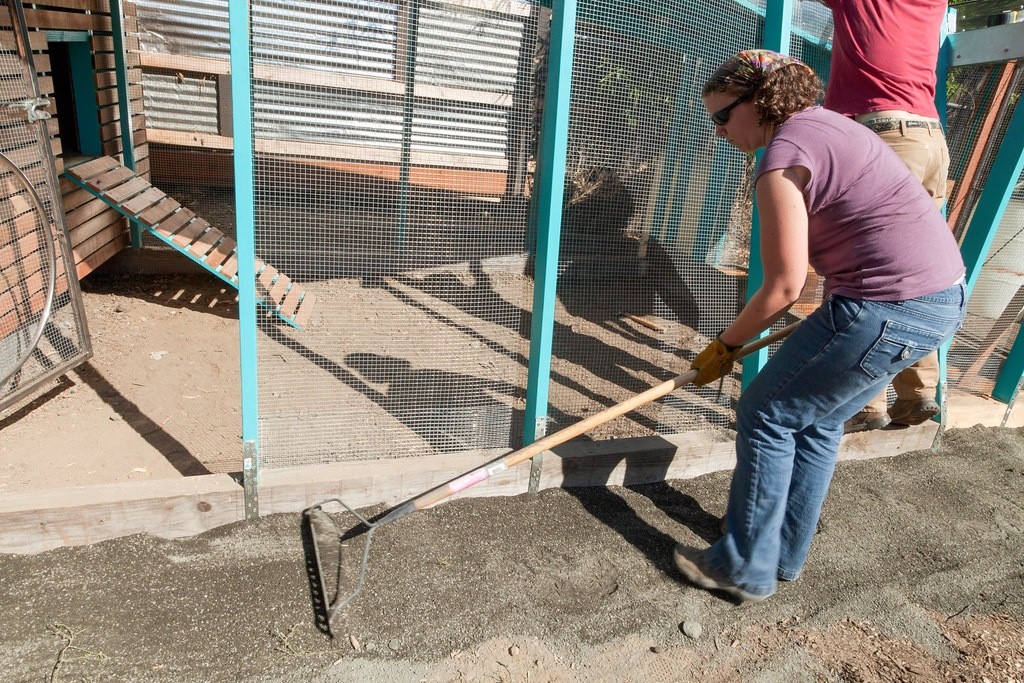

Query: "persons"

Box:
675,49,968,600
822,0,950,432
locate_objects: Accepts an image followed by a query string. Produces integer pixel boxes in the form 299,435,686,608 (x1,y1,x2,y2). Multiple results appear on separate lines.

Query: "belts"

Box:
866,120,942,132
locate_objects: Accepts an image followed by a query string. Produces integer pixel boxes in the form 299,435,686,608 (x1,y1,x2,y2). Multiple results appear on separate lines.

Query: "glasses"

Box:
712,93,749,126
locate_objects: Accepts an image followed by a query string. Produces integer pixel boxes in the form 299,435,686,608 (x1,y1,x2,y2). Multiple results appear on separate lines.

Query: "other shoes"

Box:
844,411,891,432
887,398,940,425
673,544,773,602
